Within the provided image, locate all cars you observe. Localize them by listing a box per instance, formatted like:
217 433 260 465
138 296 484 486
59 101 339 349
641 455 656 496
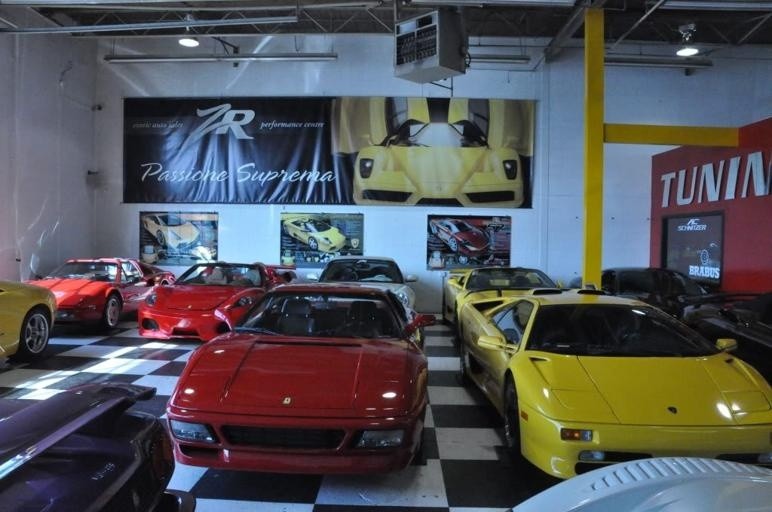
165 277 440 479
428 216 509 268
0 279 57 359
282 216 361 266
0 373 196 512
140 212 216 264
138 262 286 343
308 255 419 310
350 116 526 209
25 258 176 333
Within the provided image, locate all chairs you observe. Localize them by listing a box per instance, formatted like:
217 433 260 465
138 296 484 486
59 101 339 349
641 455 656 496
204 269 227 284
369 266 387 276
511 276 531 286
245 262 268 285
271 298 317 334
472 274 490 288
334 301 385 338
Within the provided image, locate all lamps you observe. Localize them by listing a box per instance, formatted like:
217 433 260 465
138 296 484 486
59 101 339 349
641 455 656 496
471 55 530 63
675 24 699 57
178 14 200 47
604 59 711 69
100 55 339 62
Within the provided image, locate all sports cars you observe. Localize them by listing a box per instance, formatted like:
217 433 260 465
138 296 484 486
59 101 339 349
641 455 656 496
461 285 772 483
672 291 772 387
567 267 708 319
442 265 563 331
